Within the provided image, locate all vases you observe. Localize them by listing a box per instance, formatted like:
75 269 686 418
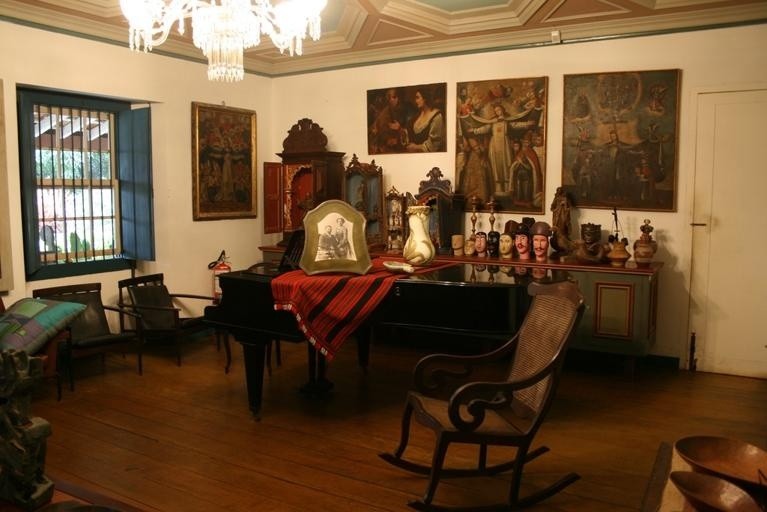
402 206 435 266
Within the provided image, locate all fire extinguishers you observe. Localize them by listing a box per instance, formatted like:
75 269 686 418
208 249 231 305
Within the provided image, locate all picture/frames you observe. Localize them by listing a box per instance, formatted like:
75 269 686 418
562 69 682 212
454 76 549 215
192 100 257 221
297 198 372 276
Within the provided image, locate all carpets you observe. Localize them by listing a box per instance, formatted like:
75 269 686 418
639 441 696 511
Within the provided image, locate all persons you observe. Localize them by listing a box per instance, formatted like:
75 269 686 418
197 107 253 212
388 88 445 152
455 80 543 211
499 234 515 258
334 217 354 260
565 73 674 210
475 232 487 256
579 223 601 253
550 187 572 237
318 225 339 261
475 263 548 283
513 223 533 259
369 88 417 154
529 222 551 262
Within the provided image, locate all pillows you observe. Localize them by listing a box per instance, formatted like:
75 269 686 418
0 298 88 356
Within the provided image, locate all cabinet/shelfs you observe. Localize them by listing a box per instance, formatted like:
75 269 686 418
264 118 347 246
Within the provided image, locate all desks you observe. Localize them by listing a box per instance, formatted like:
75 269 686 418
258 245 664 357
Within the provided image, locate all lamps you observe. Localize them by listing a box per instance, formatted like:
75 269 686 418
123 0 327 84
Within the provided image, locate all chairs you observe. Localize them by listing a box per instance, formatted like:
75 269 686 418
377 282 587 510
32 282 148 390
0 291 67 400
118 273 223 365
